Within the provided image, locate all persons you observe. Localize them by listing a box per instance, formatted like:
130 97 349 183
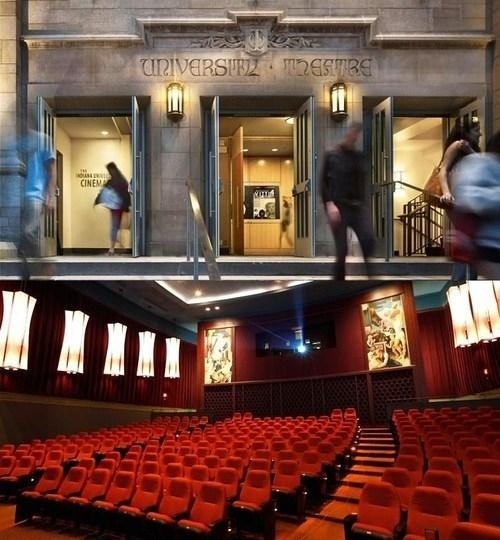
280 195 293 248
0 102 56 258
104 162 130 255
255 202 275 219
321 120 378 282
440 117 500 280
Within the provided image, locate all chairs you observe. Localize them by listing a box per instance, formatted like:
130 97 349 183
1 407 500 540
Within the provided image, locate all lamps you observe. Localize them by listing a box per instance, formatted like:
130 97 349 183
164 337 182 379
0 290 38 373
446 278 500 350
102 323 128 376
136 330 157 379
56 310 90 375
166 83 185 121
330 83 347 122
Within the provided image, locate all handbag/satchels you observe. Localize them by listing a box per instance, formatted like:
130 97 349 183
423 167 454 210
120 211 131 230
